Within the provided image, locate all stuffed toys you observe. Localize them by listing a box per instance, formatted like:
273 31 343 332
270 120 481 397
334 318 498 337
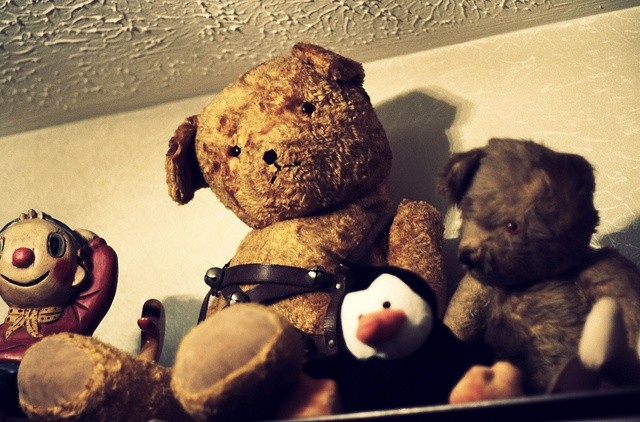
1 209 119 420
292 244 520 421
18 43 444 420
446 136 640 394
